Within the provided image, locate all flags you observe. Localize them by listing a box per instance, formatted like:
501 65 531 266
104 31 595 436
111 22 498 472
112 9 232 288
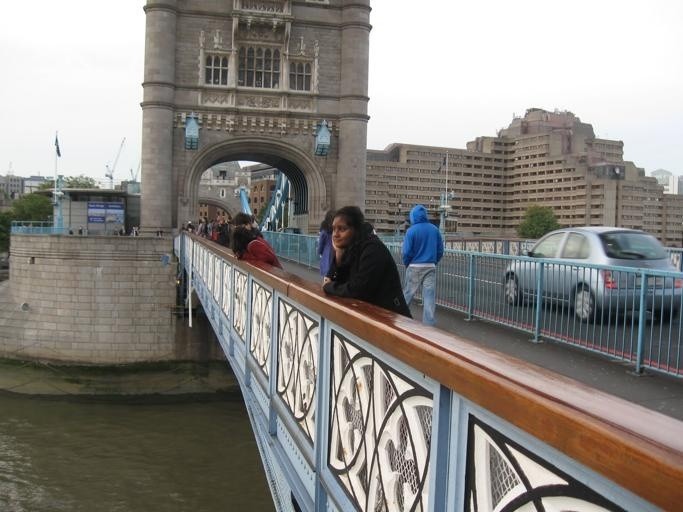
436 157 446 173
55 137 62 158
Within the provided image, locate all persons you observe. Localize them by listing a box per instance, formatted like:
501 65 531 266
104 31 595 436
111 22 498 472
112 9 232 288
402 205 444 326
322 205 412 319
318 211 336 281
181 213 282 269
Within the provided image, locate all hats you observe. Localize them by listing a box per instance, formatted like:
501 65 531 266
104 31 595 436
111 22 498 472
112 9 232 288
227 212 255 226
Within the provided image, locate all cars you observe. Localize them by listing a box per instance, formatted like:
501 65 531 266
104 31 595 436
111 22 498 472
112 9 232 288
274 227 307 253
501 226 683 322
